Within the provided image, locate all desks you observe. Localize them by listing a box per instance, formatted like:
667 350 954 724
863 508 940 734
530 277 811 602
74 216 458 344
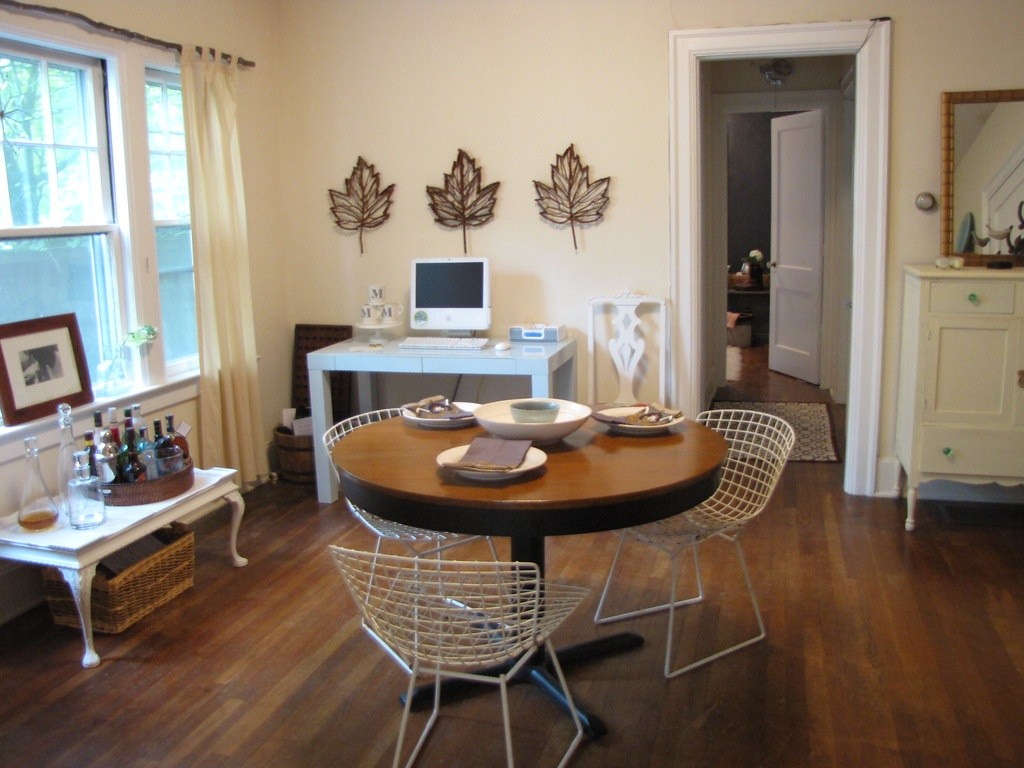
306 336 578 504
331 403 729 741
328 545 584 767
0 467 248 668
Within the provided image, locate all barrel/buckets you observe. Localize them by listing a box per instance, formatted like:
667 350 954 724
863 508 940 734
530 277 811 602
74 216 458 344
273 426 316 483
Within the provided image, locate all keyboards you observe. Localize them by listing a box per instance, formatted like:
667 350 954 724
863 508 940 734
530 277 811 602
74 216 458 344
399 337 489 350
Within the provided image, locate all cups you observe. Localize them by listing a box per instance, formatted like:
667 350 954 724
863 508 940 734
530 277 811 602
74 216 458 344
144 447 184 479
360 285 403 325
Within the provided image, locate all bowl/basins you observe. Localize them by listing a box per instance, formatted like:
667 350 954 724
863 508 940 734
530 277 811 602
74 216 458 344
473 397 593 445
510 400 560 423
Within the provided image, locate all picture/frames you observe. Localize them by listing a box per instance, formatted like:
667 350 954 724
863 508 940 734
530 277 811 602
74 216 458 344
0 313 94 426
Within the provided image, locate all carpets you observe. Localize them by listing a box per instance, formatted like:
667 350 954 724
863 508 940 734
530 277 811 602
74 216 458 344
705 399 842 463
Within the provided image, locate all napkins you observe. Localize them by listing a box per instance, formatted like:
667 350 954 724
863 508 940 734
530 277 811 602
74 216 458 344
590 404 682 425
401 395 473 420
442 436 532 471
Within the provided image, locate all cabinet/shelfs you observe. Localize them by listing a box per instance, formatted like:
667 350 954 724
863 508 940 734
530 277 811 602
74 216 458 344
893 264 1024 531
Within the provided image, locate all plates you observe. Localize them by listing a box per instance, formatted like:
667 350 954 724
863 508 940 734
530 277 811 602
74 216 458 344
607 407 685 433
436 444 547 480
402 401 482 428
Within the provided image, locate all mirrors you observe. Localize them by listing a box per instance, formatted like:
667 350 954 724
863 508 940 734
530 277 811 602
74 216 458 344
940 89 1024 267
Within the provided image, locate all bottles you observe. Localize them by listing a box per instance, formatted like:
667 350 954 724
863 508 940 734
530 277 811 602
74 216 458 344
57 405 80 515
65 453 106 529
17 437 59 530
84 403 190 484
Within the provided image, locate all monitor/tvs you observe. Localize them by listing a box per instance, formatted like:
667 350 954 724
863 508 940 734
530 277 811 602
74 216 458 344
410 257 492 338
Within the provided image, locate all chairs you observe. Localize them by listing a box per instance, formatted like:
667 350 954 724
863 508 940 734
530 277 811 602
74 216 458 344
323 408 514 675
586 295 671 409
594 408 797 679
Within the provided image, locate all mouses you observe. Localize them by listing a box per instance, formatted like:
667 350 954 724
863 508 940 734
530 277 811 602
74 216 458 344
495 343 510 351
